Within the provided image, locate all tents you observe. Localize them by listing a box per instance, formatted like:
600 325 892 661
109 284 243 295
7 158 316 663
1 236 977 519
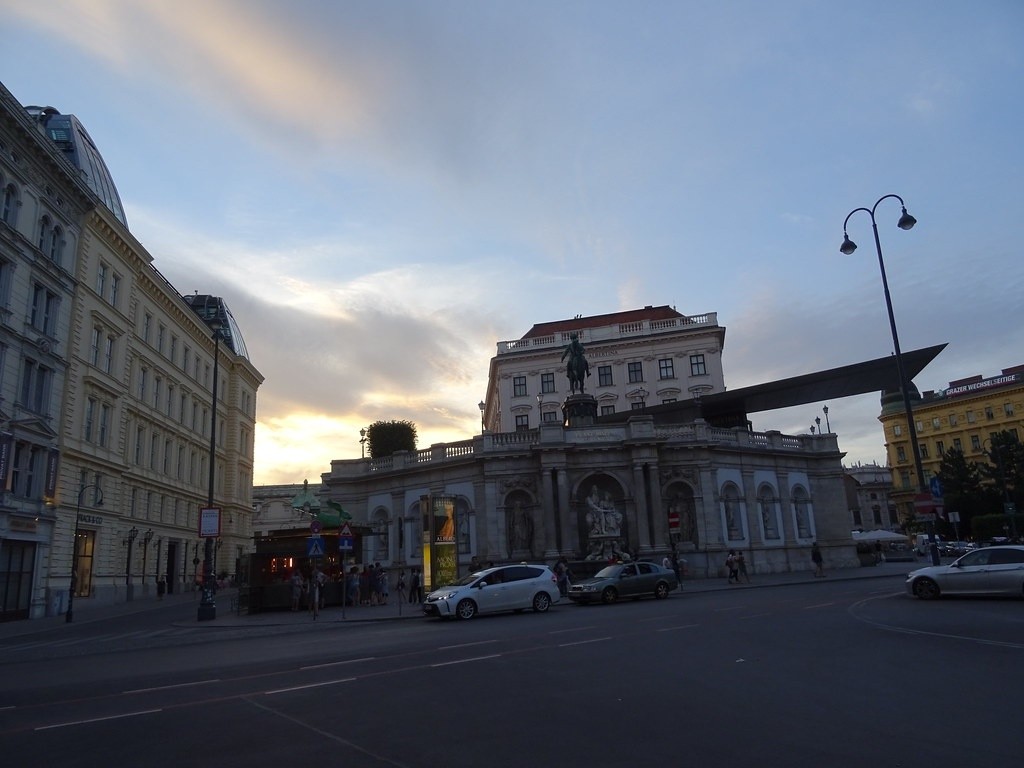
853 528 909 542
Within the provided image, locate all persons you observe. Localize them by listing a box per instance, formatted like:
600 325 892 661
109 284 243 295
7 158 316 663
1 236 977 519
290 561 423 611
609 555 618 566
554 557 575 597
811 541 826 578
919 541 933 562
873 540 884 568
726 550 753 585
631 548 641 564
585 485 623 535
561 334 591 380
671 554 687 591
468 556 482 572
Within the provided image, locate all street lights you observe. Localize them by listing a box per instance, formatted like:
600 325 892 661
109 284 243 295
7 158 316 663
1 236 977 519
66 486 105 623
360 428 366 458
197 312 226 622
536 392 544 422
478 401 486 434
837 192 943 565
810 405 830 435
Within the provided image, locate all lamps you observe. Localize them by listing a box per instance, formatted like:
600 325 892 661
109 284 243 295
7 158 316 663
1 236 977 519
129 526 138 540
145 529 154 543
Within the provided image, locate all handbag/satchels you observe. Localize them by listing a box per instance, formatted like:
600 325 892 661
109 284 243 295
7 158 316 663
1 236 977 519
554 565 561 576
726 560 729 566
401 582 404 589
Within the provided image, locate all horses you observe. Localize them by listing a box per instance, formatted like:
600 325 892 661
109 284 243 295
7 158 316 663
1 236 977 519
568 348 586 395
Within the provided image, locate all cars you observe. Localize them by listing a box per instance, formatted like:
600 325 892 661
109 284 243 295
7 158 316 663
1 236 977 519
566 560 678 604
423 564 562 620
912 534 1010 557
904 545 1024 601
